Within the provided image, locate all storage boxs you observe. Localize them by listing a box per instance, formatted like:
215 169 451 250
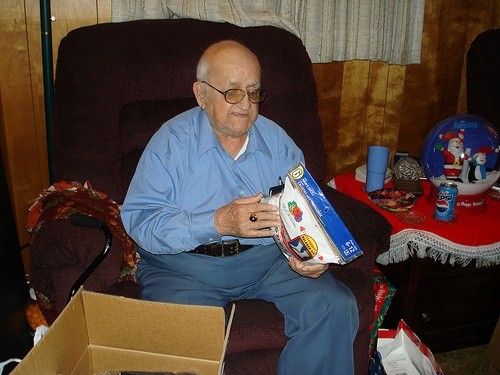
11 284 234 375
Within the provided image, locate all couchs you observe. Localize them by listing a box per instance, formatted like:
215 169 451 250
30 20 391 373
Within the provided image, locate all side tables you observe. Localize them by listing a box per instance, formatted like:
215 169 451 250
327 172 500 352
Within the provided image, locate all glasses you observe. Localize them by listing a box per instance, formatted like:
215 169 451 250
202 81 268 104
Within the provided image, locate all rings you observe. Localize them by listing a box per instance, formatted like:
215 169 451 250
249 212 257 223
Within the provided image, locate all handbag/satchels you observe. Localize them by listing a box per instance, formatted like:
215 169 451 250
376 319 443 375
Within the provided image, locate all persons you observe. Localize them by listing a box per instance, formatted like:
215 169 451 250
436 131 476 183
120 40 360 375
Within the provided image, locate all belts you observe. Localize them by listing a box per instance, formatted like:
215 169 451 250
185 240 254 258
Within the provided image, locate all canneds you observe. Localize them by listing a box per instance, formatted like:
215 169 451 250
435 182 458 221
